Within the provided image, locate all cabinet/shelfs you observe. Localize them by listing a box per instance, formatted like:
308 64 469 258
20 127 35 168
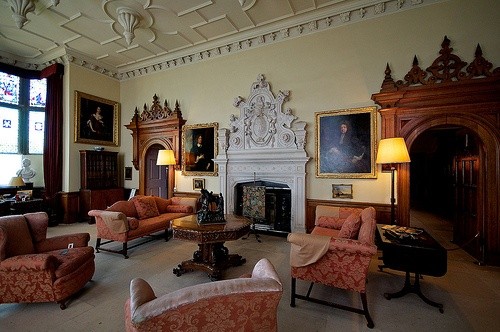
80 150 126 225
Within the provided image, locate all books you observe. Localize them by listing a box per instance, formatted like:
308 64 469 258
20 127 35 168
87 153 118 189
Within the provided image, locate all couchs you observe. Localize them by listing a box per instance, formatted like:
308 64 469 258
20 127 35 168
124 257 284 332
0 211 95 310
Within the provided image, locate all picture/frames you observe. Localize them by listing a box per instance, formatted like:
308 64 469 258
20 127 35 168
73 90 119 148
332 184 353 199
314 105 378 179
192 178 205 191
182 122 219 177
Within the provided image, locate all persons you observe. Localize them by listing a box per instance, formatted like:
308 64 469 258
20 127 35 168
86 105 106 137
16 158 38 183
189 134 211 170
326 120 369 174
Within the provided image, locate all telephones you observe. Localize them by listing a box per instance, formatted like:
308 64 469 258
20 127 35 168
384 229 411 244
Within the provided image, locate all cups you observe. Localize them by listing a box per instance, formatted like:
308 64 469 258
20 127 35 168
16 194 19 201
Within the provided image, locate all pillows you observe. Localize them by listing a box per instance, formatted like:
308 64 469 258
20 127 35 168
127 216 139 228
166 204 193 213
132 196 160 219
339 211 361 238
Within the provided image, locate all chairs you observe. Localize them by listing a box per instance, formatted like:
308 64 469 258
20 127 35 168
0 201 10 217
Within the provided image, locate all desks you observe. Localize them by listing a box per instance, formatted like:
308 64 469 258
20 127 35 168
170 213 253 282
0 198 44 216
376 222 448 314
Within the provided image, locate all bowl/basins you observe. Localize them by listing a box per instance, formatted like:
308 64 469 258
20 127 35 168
95 147 104 151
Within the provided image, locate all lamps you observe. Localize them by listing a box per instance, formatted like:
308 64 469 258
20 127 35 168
156 149 176 200
8 177 26 194
375 137 411 225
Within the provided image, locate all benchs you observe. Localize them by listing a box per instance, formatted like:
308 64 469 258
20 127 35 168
88 193 197 261
286 204 377 329
